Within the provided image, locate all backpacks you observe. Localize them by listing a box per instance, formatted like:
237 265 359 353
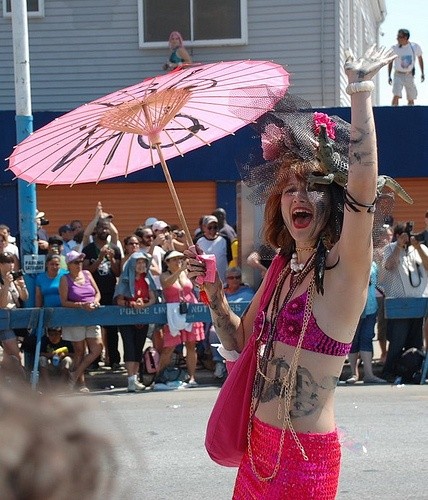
400 347 426 385
140 346 170 386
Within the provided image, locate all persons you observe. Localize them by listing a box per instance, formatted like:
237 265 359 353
163 32 192 72
0 201 427 392
0 373 119 499
387 29 425 106
183 41 398 500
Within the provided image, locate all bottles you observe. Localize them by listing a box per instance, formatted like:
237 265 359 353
52 353 60 367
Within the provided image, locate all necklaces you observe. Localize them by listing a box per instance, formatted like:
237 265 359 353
247 246 328 483
289 253 304 272
295 247 314 252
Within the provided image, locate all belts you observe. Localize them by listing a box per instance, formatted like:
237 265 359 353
395 70 412 74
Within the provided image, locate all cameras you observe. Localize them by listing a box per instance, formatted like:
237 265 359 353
405 221 426 245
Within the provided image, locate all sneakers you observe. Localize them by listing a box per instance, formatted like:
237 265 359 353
214 363 225 378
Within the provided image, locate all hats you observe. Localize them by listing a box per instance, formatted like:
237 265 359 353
152 221 169 232
36 209 45 218
228 83 413 258
202 215 218 225
101 212 112 219
58 225 76 234
66 251 85 264
164 251 185 262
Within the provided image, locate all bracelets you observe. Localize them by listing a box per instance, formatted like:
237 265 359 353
346 81 375 96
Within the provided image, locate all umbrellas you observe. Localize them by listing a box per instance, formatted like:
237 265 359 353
4 59 294 280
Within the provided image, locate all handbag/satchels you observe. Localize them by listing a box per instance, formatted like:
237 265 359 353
205 309 267 467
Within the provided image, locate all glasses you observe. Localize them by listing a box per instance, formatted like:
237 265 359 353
206 225 218 230
2 252 11 257
71 261 84 264
142 235 154 239
128 242 139 245
226 276 241 279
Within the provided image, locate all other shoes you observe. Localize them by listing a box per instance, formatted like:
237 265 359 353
66 351 206 393
346 376 358 384
364 376 387 383
380 375 396 383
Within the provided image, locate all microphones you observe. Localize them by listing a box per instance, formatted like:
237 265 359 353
10 268 25 281
